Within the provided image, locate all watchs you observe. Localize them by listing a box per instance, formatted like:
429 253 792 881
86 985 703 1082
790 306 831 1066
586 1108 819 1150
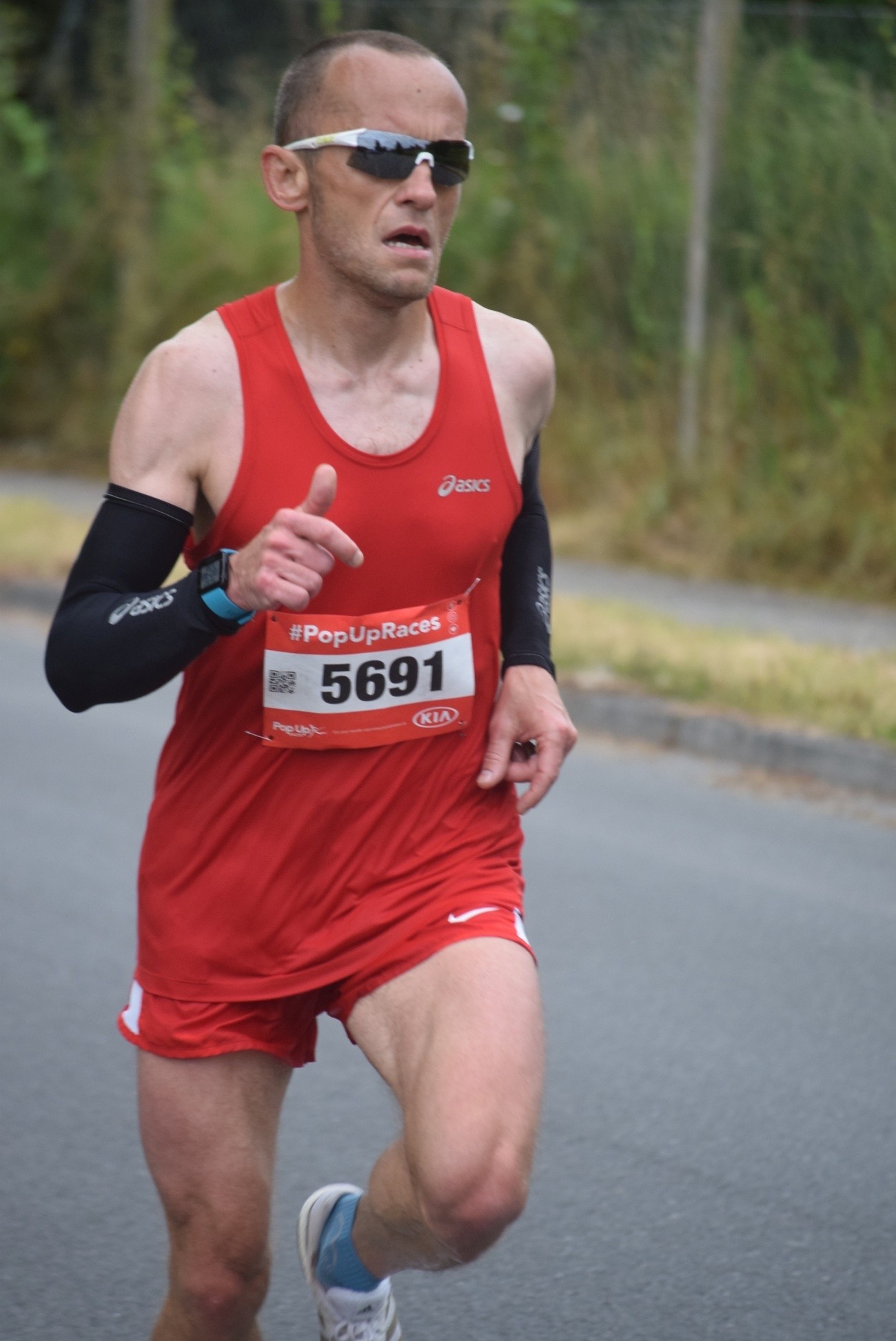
198 547 256 626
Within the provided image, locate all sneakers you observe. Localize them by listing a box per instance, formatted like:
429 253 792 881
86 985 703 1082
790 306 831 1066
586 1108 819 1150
297 1182 403 1341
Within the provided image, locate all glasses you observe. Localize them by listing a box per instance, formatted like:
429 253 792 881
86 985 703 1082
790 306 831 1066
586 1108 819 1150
284 128 474 186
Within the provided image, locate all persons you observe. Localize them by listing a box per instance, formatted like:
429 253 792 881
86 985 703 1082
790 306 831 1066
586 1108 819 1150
42 30 577 1341
